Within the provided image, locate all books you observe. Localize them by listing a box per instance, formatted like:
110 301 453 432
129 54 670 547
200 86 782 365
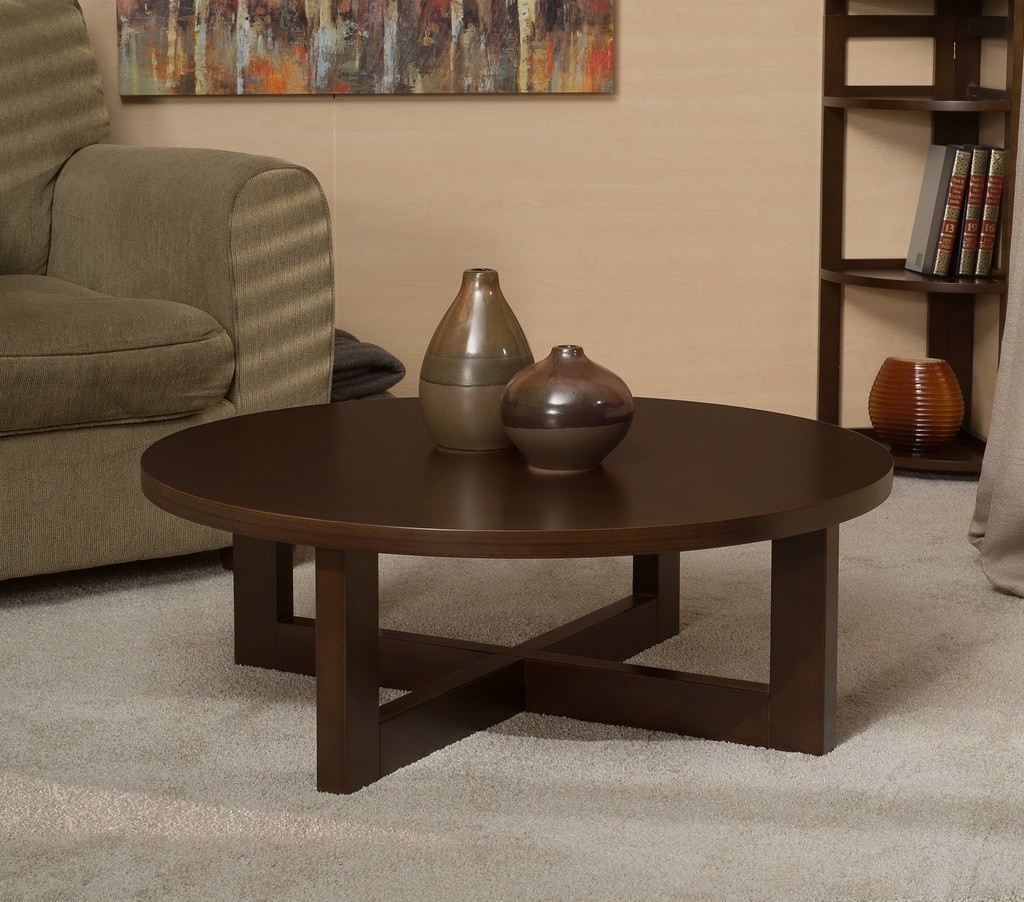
905 144 1006 279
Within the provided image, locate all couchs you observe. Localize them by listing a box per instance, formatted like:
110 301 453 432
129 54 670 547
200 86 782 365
0 0 336 584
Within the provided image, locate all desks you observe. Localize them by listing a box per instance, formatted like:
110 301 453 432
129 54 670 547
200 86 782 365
142 398 894 798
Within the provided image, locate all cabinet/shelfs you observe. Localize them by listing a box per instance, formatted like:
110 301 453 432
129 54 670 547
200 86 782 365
820 1 1023 473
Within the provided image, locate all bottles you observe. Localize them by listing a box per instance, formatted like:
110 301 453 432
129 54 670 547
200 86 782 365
420 268 535 451
501 345 635 471
